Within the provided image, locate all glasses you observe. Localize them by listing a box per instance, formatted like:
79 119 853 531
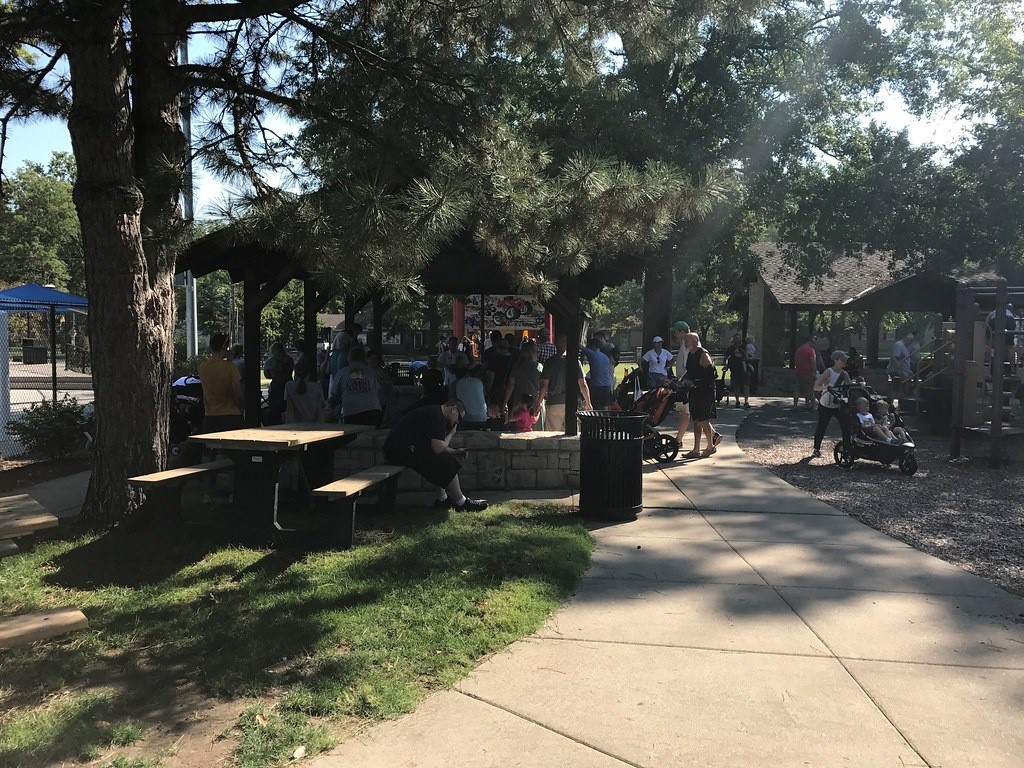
657 355 660 364
450 404 463 424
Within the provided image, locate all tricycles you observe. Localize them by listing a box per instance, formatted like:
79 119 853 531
715 369 730 407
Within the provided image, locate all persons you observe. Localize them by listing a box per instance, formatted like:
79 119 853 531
196 322 758 510
885 333 915 400
382 395 488 511
791 332 922 457
813 350 855 457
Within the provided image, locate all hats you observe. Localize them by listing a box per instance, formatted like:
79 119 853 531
653 336 664 342
831 350 850 360
669 321 690 331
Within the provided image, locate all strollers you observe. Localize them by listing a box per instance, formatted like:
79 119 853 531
615 367 644 411
625 378 693 463
828 383 919 476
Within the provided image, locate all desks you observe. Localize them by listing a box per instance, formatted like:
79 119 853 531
0 494 59 540
187 422 375 535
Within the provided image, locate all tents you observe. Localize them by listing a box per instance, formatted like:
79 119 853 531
0 284 88 312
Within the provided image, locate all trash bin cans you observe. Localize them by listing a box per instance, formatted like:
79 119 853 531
20 339 48 364
576 410 649 519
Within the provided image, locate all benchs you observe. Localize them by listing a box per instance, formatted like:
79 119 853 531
311 465 407 546
0 605 88 649
0 539 20 559
127 458 238 515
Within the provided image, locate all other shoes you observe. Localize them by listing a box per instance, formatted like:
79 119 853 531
891 436 903 444
682 451 700 458
712 433 723 445
813 449 822 457
446 495 487 512
668 439 682 448
701 446 716 457
